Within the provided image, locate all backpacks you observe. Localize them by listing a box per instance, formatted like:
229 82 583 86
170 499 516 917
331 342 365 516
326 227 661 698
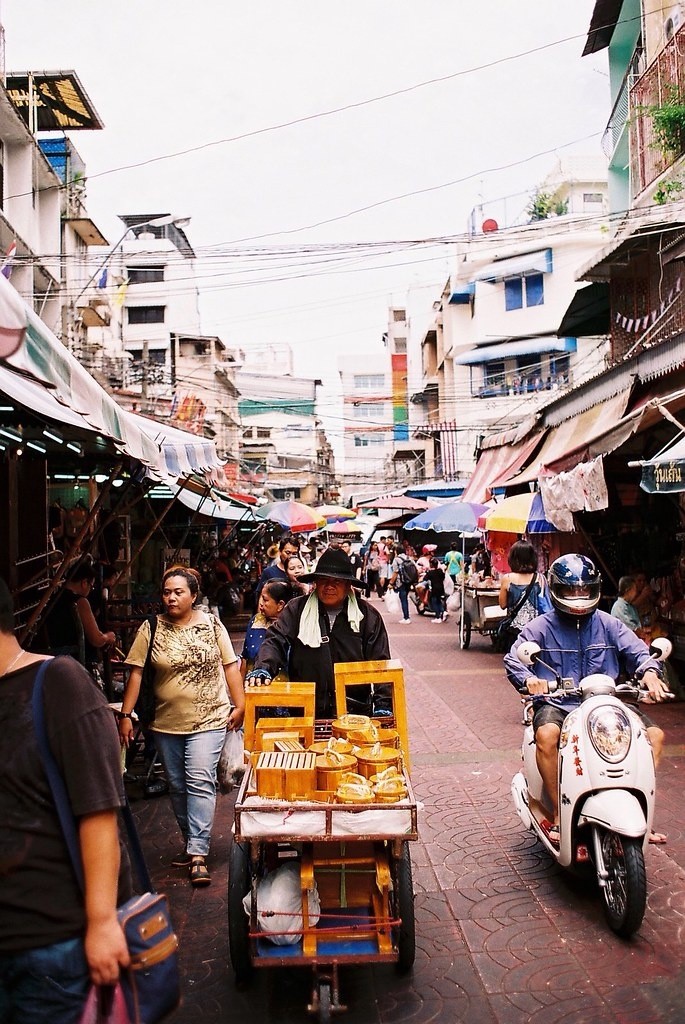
397 556 418 584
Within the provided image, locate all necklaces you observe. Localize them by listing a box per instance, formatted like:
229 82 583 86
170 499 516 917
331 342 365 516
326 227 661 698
3 650 25 675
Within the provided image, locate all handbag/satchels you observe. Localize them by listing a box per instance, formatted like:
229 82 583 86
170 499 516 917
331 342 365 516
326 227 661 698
443 571 454 595
217 728 246 795
133 615 157 724
495 617 515 652
117 892 182 1024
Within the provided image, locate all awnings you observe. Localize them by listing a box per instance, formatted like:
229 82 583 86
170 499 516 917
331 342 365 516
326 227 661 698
460 379 685 506
0 269 265 636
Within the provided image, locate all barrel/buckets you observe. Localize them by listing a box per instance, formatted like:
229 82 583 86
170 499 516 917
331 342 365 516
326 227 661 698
309 737 358 791
332 715 401 787
336 785 408 804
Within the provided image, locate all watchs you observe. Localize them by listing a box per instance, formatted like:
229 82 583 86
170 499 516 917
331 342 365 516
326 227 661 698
118 712 131 720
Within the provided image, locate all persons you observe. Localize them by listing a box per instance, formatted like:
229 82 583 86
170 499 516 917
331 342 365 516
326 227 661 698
117 563 245 884
502 553 669 844
203 536 491 625
42 561 116 671
0 583 131 1024
611 572 656 632
243 546 393 727
499 541 552 726
238 578 304 683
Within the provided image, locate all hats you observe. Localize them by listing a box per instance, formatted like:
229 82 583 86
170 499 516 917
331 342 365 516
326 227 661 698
296 548 369 589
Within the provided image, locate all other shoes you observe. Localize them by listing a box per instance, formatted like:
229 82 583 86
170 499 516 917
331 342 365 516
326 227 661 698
638 693 656 704
191 860 211 882
431 617 442 623
399 619 410 624
172 848 193 865
443 611 448 621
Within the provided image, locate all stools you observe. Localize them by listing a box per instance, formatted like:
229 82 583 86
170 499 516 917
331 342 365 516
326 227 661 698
255 716 317 757
333 659 410 775
241 680 315 768
294 840 393 957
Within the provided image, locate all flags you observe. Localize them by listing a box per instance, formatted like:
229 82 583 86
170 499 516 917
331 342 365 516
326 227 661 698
616 278 681 333
99 268 107 289
114 281 126 304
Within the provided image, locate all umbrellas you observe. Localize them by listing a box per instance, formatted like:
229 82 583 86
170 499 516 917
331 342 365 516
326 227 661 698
477 489 565 572
257 494 434 543
462 519 493 650
403 501 492 650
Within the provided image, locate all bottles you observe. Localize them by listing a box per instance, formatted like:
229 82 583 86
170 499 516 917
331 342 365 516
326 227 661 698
472 572 479 588
485 576 491 588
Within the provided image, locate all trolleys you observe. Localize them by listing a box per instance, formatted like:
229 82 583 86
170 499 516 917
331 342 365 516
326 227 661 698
454 582 508 650
227 692 418 1024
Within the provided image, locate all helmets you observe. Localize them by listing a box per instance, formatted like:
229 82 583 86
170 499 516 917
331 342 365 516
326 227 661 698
547 554 601 615
422 544 437 553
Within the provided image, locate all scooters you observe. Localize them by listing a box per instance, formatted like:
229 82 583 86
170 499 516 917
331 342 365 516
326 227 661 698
415 580 450 618
518 637 676 936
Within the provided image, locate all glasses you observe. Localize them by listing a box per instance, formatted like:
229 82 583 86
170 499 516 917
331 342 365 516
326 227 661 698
87 580 95 592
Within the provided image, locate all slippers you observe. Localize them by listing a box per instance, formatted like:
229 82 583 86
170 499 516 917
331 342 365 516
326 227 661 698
648 829 666 843
549 827 561 845
